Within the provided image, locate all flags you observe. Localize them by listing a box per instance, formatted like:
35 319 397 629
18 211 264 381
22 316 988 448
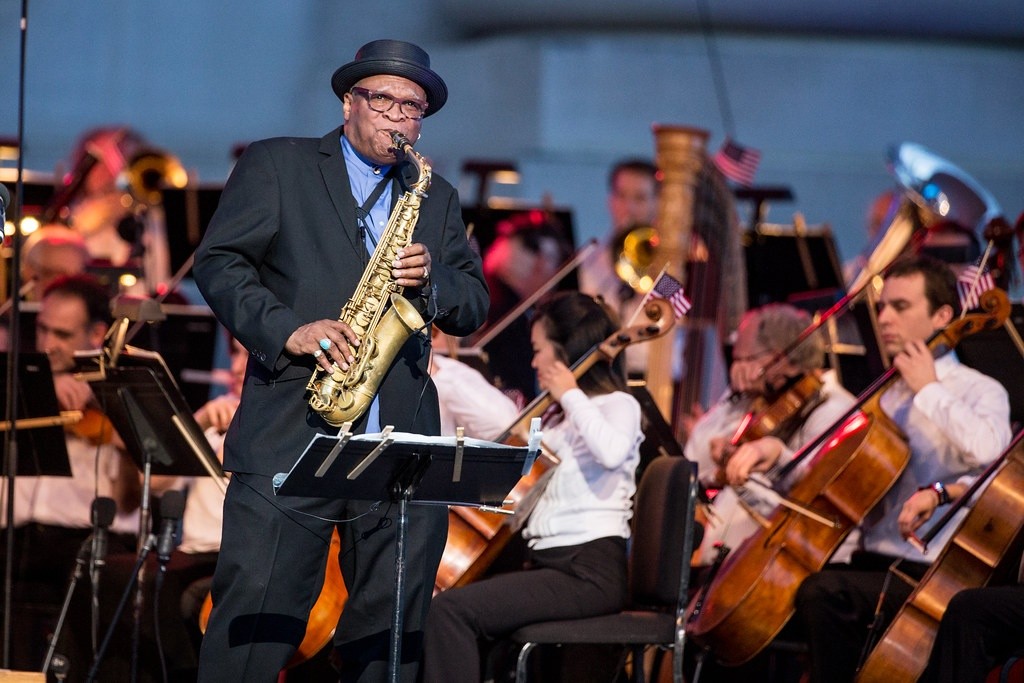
645 272 694 319
956 256 996 311
713 137 760 186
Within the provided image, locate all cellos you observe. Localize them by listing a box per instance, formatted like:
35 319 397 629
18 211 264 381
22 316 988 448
686 285 1012 664
847 423 1024 683
200 526 349 665
430 292 680 604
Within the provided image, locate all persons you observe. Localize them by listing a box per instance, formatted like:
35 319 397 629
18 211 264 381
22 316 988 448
199 39 490 683
0 129 254 675
682 303 859 592
575 160 706 384
423 291 646 683
428 353 520 442
726 257 1012 683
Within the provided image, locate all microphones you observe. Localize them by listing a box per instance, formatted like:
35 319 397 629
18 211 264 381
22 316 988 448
89 497 117 593
156 490 186 565
0 182 11 245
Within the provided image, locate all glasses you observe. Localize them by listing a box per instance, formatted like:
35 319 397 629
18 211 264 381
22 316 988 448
349 86 429 119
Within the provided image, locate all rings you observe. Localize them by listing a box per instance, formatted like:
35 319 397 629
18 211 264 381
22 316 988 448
314 349 322 358
422 266 429 279
320 338 331 349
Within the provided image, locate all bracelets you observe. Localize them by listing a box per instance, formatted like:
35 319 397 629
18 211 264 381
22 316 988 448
930 481 951 506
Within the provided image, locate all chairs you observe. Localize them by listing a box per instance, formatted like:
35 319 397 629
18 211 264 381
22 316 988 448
486 451 699 683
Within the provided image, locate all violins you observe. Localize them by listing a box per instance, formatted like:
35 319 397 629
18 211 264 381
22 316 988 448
66 393 120 448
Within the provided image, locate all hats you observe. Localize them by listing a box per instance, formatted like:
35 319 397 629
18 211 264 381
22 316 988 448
332 39 447 119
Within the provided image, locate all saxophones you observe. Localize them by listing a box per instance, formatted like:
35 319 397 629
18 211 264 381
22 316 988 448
306 125 436 428
